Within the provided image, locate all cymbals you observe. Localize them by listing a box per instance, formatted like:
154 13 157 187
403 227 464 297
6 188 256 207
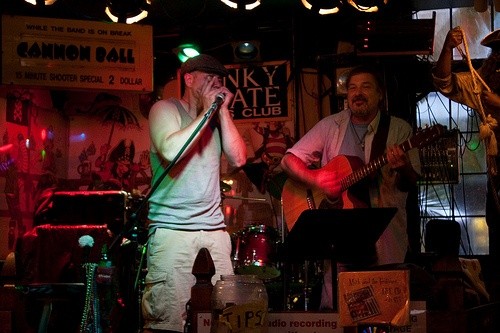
267 167 317 201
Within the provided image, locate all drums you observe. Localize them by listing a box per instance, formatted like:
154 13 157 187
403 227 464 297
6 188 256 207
234 223 283 280
229 228 248 261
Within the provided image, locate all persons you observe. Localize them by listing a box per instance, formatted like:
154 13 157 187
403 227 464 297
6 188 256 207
281 66 422 310
432 26 500 254
142 54 248 333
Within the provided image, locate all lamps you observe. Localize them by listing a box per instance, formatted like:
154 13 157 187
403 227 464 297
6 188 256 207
98 0 396 64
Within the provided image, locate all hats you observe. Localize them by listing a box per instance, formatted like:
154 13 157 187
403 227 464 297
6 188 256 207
180 54 231 75
480 30 500 47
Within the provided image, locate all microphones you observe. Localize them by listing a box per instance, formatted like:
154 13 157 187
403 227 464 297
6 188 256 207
215 93 225 105
78 235 94 264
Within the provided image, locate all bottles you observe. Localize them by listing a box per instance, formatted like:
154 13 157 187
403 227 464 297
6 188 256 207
212 274 269 314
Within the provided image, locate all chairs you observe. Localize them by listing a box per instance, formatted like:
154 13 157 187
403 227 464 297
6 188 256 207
13 223 117 333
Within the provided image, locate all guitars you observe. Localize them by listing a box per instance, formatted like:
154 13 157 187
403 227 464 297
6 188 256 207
281 122 448 234
